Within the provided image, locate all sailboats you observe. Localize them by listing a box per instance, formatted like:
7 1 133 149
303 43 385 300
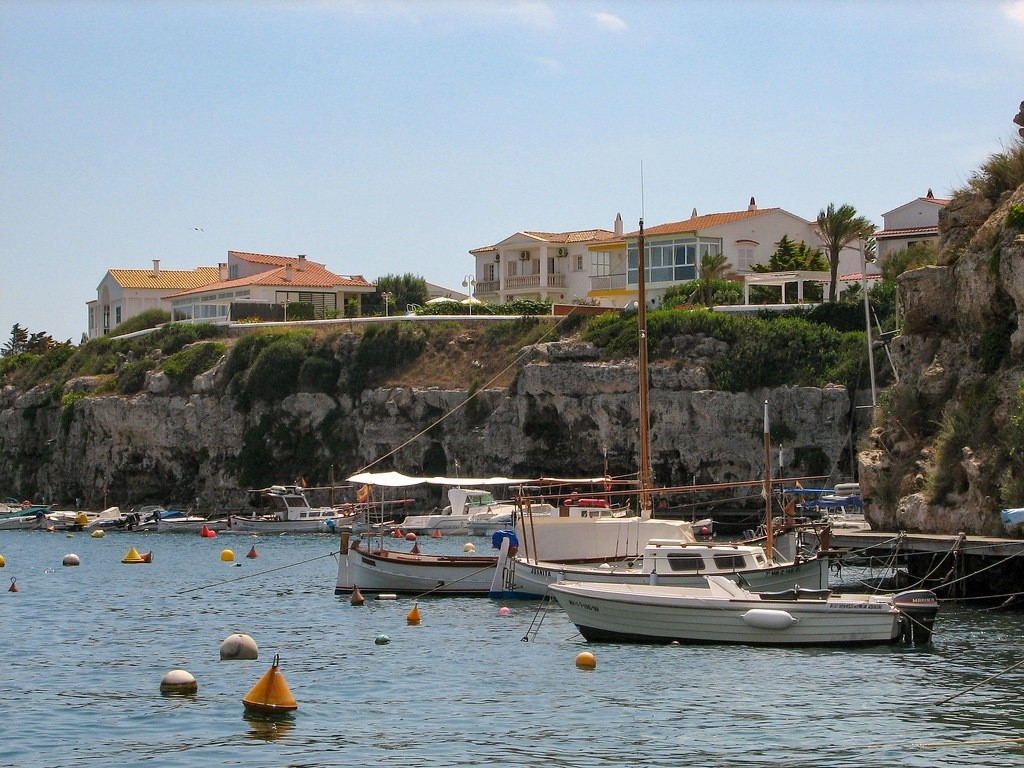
330 216 864 602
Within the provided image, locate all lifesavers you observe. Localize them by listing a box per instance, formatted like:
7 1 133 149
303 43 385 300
492 530 519 558
22 501 31 510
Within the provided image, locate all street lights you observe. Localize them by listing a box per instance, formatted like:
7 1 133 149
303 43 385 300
381 291 394 317
462 274 477 316
282 299 290 322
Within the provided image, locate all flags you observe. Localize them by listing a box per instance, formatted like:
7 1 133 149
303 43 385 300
356 484 369 503
302 479 306 488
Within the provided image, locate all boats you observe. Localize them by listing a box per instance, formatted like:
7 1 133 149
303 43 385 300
0 496 231 535
546 399 942 650
228 484 368 537
